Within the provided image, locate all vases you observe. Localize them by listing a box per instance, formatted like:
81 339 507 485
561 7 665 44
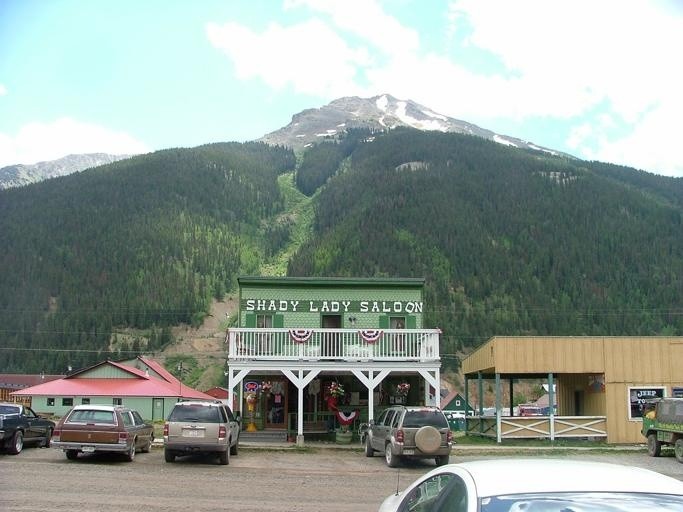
326 397 337 410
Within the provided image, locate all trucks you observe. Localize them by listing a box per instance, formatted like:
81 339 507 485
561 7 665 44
639 394 683 462
518 402 545 418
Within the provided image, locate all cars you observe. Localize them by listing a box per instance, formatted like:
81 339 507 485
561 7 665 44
374 456 683 512
53 402 156 462
0 402 56 455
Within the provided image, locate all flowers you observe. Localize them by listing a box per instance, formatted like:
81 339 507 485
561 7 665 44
329 382 345 399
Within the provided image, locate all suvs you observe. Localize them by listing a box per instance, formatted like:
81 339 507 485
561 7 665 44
362 402 456 468
161 396 243 468
